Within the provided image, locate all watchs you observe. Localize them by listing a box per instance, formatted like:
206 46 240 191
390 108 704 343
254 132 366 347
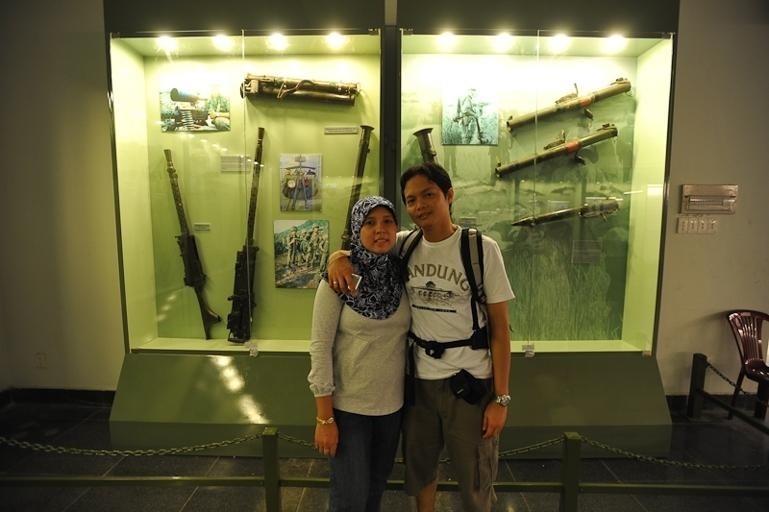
492 391 513 408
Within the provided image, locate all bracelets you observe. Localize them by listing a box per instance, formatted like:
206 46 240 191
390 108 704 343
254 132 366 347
315 415 335 424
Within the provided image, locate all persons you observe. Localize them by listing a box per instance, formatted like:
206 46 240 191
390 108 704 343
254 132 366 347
316 158 517 510
274 224 328 274
302 192 410 512
458 86 484 145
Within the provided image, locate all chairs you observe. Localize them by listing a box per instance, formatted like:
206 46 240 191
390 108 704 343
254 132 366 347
726 310 769 421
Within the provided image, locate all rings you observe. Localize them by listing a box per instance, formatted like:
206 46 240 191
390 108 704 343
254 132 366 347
333 281 338 284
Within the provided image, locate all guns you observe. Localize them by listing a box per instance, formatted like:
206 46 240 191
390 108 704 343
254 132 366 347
164 149 220 339
228 127 264 343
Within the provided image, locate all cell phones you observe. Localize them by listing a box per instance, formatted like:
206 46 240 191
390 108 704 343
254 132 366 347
348 274 362 290
